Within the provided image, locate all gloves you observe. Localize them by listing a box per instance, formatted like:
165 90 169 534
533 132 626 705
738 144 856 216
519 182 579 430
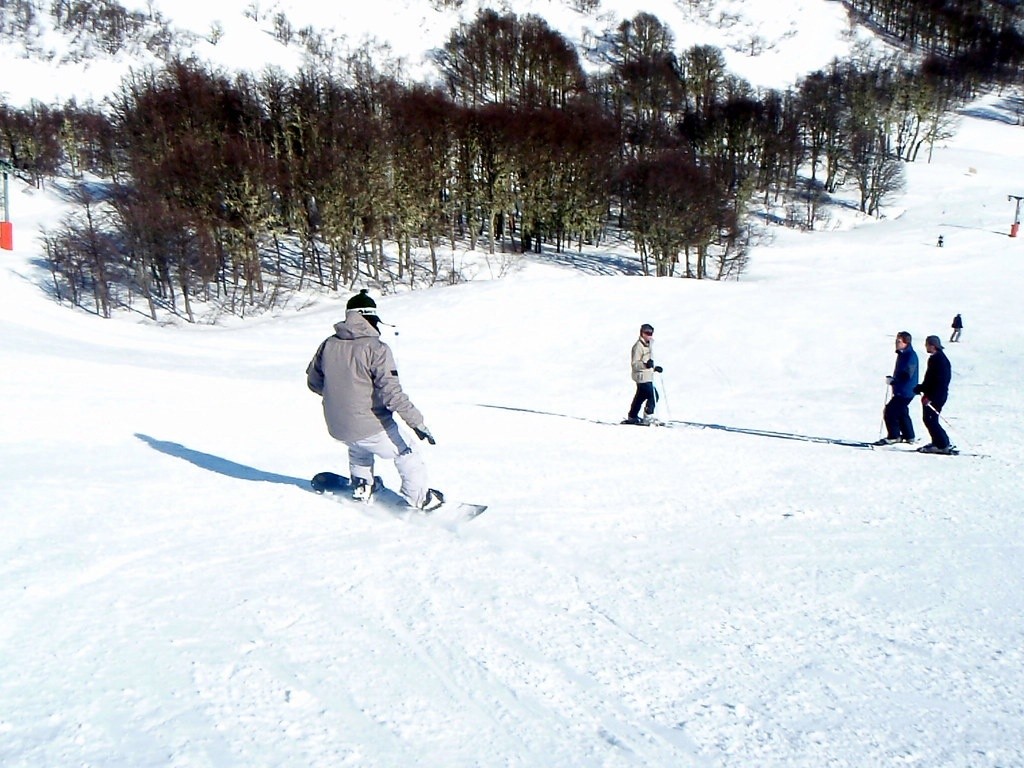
921 396 931 405
886 377 894 385
646 359 653 368
412 422 436 446
913 384 922 394
654 366 662 373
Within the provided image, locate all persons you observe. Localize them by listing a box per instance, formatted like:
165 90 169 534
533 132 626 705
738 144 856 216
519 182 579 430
628 324 664 426
913 335 951 453
949 314 963 342
305 289 444 513
879 331 919 445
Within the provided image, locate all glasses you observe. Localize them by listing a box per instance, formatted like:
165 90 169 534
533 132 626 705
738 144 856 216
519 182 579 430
643 330 653 336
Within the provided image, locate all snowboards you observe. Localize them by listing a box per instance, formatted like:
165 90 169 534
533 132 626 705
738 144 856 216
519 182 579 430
309 470 488 526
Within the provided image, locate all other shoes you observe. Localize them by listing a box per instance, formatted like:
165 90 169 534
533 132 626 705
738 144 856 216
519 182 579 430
955 340 959 342
949 340 954 342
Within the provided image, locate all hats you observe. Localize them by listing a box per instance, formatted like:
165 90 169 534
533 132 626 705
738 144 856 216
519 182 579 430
925 336 945 349
898 331 912 343
345 289 395 329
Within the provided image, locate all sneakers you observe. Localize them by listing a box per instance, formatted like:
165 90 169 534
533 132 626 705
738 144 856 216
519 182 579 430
903 437 915 445
352 475 385 502
918 444 949 454
628 414 643 425
875 436 902 445
398 490 444 510
643 409 664 426
